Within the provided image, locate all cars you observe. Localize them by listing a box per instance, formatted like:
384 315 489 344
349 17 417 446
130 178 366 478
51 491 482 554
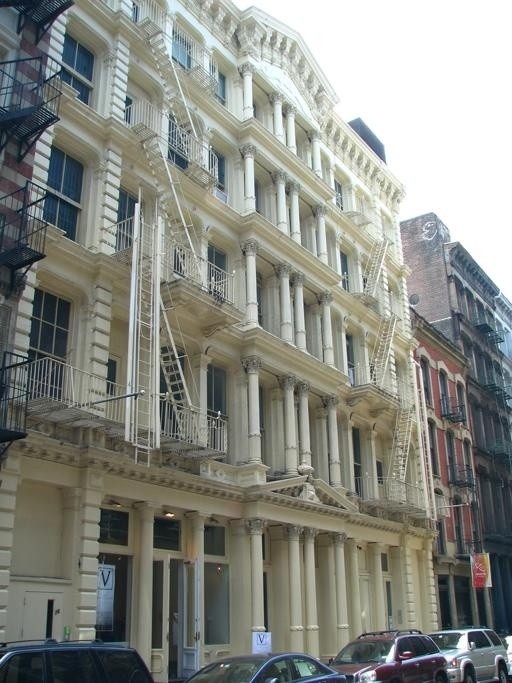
184 652 347 683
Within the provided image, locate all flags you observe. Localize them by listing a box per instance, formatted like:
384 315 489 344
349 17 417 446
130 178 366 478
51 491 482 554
469 552 495 589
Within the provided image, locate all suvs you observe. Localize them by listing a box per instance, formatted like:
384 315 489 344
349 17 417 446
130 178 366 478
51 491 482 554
322 625 512 682
0 638 156 683
